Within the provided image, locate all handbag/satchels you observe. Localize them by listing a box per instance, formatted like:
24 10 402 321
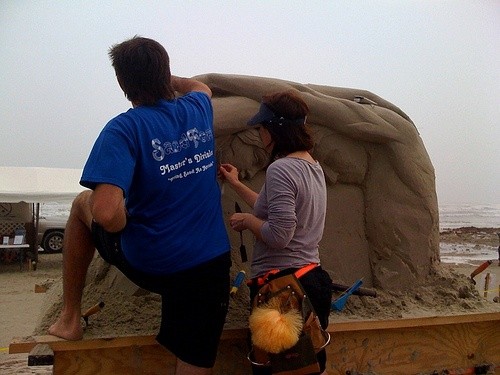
247 273 331 375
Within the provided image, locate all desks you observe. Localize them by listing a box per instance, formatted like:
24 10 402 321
0 244 29 271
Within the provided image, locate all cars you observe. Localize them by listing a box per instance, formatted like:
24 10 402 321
35 216 69 253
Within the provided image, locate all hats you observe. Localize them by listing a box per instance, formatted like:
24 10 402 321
247 92 309 127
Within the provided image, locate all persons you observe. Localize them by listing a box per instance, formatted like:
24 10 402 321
48 35 234 375
217 90 332 375
